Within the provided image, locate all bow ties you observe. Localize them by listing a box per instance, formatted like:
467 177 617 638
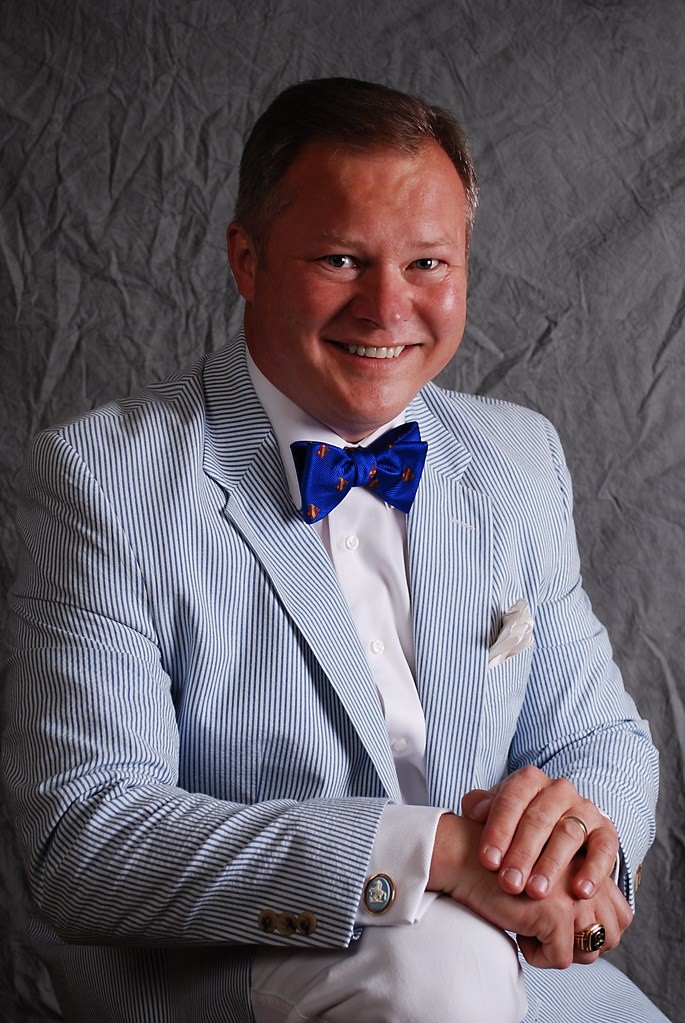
290 422 429 525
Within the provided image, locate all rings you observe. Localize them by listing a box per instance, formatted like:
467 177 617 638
561 816 588 841
574 923 605 953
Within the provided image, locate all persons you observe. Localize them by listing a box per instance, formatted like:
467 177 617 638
0 77 670 1023
